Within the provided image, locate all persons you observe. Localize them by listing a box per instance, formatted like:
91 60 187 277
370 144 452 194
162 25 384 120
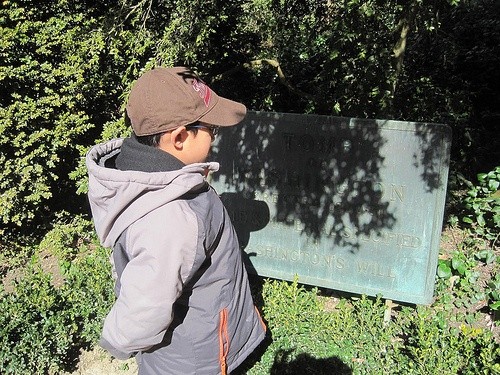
85 67 266 375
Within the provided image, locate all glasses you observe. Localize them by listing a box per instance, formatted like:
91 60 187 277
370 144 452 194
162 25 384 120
185 125 220 135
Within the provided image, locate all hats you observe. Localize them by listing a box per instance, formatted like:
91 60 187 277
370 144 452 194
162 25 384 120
127 67 246 136
